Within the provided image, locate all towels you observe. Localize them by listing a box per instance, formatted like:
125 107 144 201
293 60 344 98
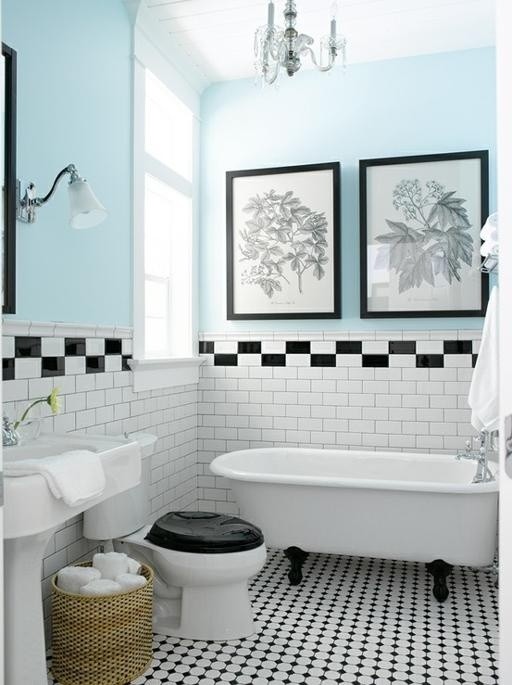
56 546 150 602
465 276 510 441
1 450 115 510
477 210 499 273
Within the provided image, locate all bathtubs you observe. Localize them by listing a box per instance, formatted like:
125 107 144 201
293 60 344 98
207 446 500 603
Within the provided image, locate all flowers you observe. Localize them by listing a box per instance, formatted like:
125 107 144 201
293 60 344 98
14 386 65 427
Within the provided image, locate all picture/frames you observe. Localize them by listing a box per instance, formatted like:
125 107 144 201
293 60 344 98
354 148 491 319
221 158 345 322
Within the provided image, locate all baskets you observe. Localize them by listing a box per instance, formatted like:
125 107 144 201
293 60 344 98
52 560 154 685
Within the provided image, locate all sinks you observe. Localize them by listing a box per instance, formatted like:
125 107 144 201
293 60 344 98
1 430 142 540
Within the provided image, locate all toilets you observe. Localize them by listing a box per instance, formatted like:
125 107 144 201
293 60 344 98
81 430 269 642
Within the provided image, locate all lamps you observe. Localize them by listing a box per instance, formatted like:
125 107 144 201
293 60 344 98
247 0 348 86
15 158 115 237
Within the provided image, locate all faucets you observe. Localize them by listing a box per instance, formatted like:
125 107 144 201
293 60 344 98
0 415 19 446
454 440 485 462
